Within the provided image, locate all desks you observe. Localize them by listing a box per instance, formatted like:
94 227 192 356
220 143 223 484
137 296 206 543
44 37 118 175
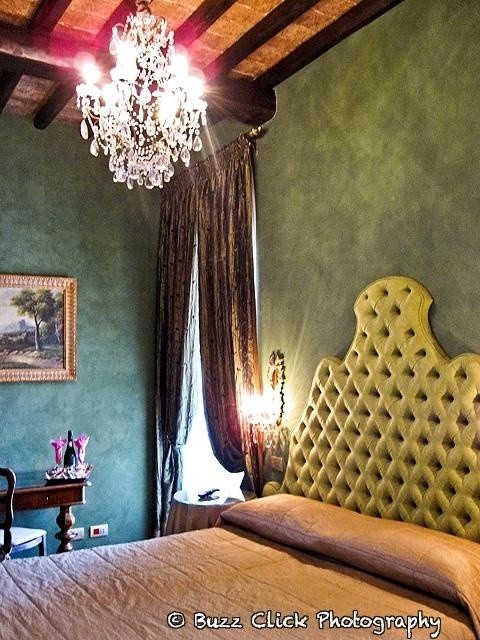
173 489 257 532
0 480 93 554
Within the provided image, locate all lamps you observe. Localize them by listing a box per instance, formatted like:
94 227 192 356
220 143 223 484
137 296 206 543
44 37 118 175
68 9 279 190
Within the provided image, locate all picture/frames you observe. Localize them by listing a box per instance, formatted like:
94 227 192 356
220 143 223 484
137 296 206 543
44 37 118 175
0 274 79 383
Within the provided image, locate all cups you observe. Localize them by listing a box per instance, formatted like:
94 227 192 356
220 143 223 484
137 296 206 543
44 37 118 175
54 447 63 465
77 446 86 465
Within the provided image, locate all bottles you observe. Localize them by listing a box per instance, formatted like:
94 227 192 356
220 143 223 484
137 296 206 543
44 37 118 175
63 430 76 468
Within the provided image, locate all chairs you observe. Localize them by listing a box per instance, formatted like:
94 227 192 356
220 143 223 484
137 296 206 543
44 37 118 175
0 466 48 561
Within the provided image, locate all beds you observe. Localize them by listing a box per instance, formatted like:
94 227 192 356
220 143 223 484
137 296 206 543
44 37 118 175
0 274 480 640
